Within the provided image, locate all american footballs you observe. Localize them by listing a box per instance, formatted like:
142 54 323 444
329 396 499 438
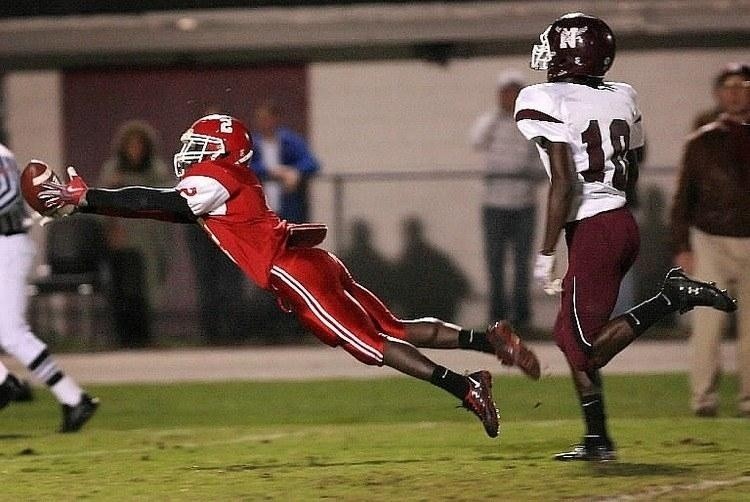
21 161 61 215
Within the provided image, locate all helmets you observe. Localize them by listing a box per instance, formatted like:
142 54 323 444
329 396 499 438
174 115 253 178
530 12 614 83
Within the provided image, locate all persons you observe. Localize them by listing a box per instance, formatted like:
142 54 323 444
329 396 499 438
669 65 750 416
241 107 321 347
513 8 740 462
0 140 100 433
38 113 539 438
179 102 246 346
94 119 175 349
470 69 552 344
686 59 738 130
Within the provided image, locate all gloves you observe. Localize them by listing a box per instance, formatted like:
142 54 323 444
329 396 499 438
34 213 65 226
37 166 89 209
535 250 562 296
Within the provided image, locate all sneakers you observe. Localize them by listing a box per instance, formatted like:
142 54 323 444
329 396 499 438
459 370 499 438
0 375 22 408
486 321 541 380
59 394 99 434
661 267 736 312
552 436 614 462
